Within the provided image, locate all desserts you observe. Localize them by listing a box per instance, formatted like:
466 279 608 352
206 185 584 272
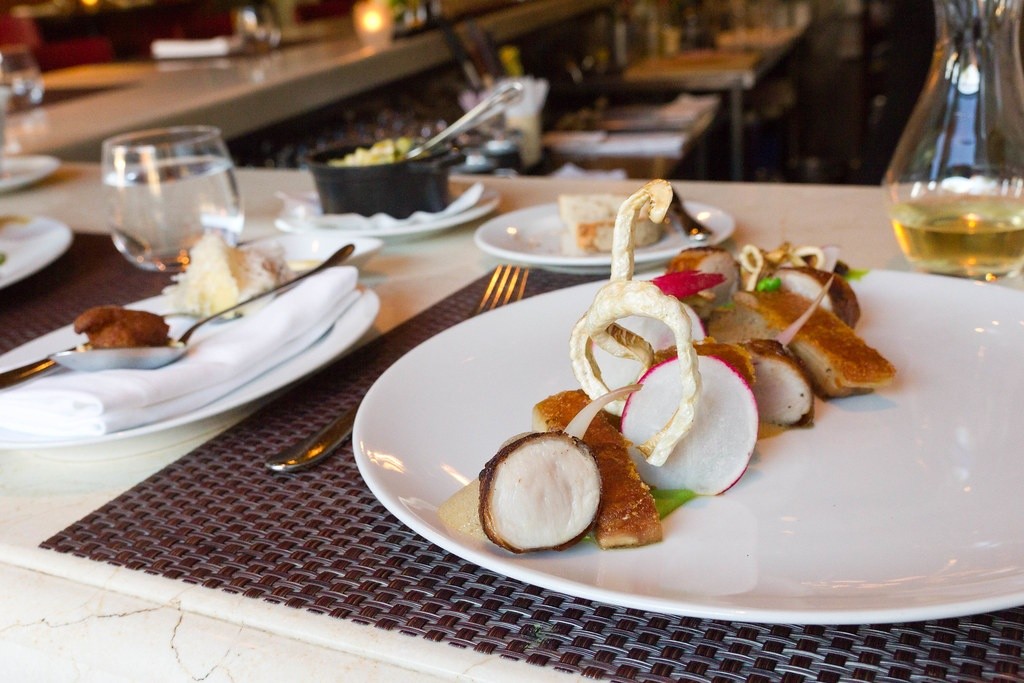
557 186 663 253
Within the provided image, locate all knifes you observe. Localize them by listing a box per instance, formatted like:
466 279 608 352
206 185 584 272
670 189 712 240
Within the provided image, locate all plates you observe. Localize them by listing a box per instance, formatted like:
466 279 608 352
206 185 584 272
240 235 384 271
1 155 60 194
0 285 382 451
274 181 500 235
0 214 74 290
353 270 1024 625
474 201 736 274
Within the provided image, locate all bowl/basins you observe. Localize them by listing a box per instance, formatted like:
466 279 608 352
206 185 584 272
304 141 465 218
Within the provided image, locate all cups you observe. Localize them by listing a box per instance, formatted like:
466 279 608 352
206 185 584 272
230 4 281 55
100 124 245 273
0 44 45 111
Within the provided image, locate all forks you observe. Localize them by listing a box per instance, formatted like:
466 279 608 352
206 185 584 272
266 265 529 470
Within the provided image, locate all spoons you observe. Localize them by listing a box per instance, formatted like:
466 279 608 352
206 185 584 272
0 312 243 389
48 244 357 371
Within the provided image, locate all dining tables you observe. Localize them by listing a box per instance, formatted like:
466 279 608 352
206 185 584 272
0 0 1024 683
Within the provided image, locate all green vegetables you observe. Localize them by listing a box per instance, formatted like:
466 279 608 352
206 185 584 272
754 267 868 292
649 488 693 519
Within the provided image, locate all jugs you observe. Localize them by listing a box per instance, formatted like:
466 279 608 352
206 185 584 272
882 0 1024 279
